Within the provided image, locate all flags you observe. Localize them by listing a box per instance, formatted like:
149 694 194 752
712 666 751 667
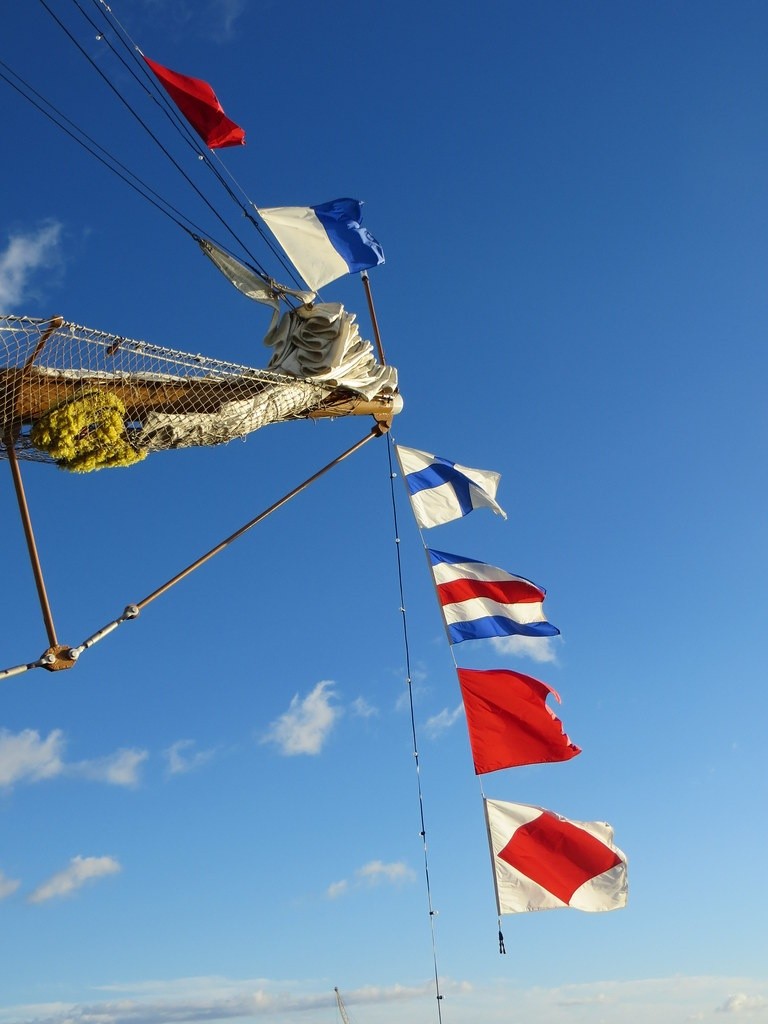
144 56 246 148
395 445 507 528
424 549 560 644
260 197 385 293
487 799 628 913
456 668 581 775
